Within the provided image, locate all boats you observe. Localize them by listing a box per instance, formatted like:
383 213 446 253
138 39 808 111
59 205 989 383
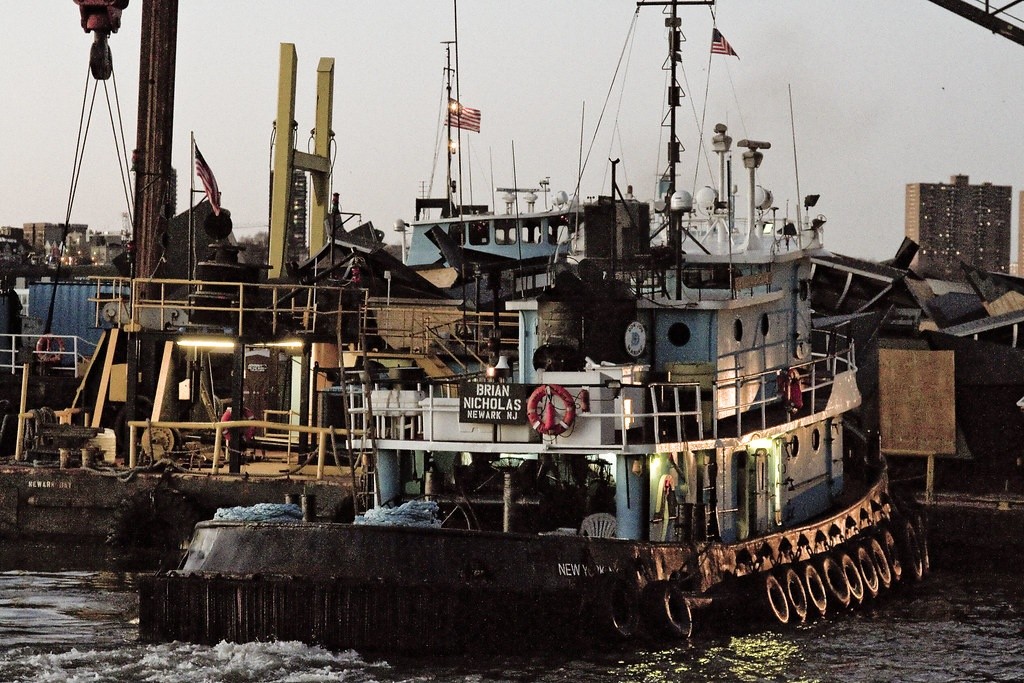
137 1 930 666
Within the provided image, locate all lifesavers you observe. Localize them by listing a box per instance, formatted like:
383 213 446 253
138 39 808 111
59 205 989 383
220 406 256 442
780 367 807 416
527 385 577 435
596 515 931 644
36 334 66 362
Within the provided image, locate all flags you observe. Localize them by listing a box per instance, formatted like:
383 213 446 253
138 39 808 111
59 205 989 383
194 143 222 217
712 28 740 59
445 99 481 133
